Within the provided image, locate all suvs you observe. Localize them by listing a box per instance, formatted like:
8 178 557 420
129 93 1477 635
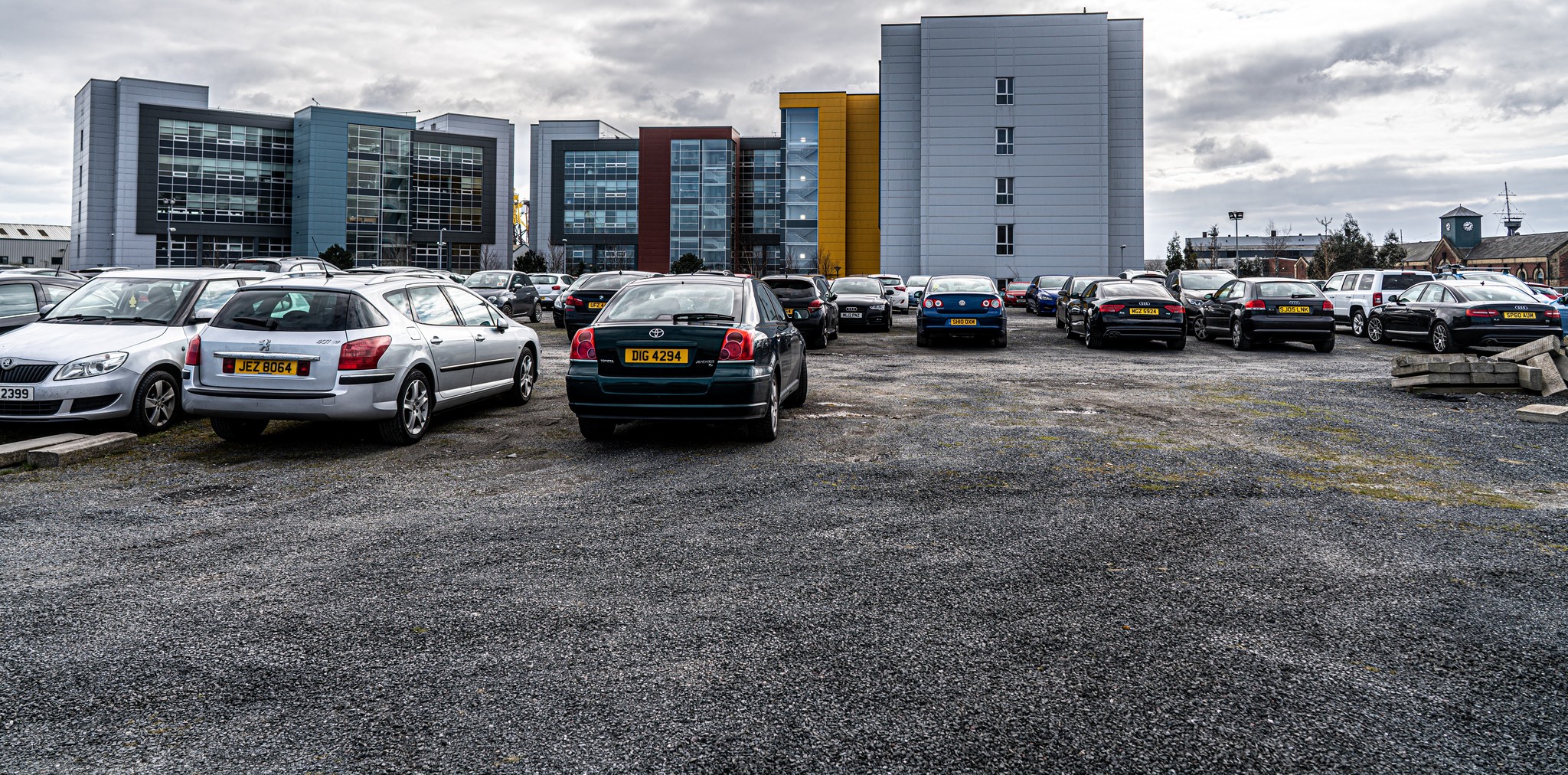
1118 268 1239 332
1321 268 1437 339
1001 281 1032 307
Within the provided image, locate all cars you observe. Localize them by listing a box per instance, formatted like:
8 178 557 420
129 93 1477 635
0 256 542 446
1192 277 1335 352
564 276 807 442
1367 267 1568 354
1055 275 1187 350
524 268 909 348
914 273 1008 348
905 275 933 307
1026 274 1074 316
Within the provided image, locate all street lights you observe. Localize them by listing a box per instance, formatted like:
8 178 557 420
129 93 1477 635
562 239 567 274
162 197 176 268
1228 210 1243 279
1120 245 1127 273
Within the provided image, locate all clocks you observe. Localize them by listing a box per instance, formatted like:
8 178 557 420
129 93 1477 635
1445 222 1451 232
1462 221 1474 232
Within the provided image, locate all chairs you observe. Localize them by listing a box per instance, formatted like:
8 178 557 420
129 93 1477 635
54 291 70 304
1277 283 1291 297
708 298 725 314
1465 289 1512 301
135 286 177 319
632 296 681 316
1076 281 1086 292
912 281 918 285
1386 279 1413 288
1434 292 1449 302
542 278 549 283
971 283 984 289
485 278 494 285
608 279 622 288
891 280 897 285
938 283 949 292
